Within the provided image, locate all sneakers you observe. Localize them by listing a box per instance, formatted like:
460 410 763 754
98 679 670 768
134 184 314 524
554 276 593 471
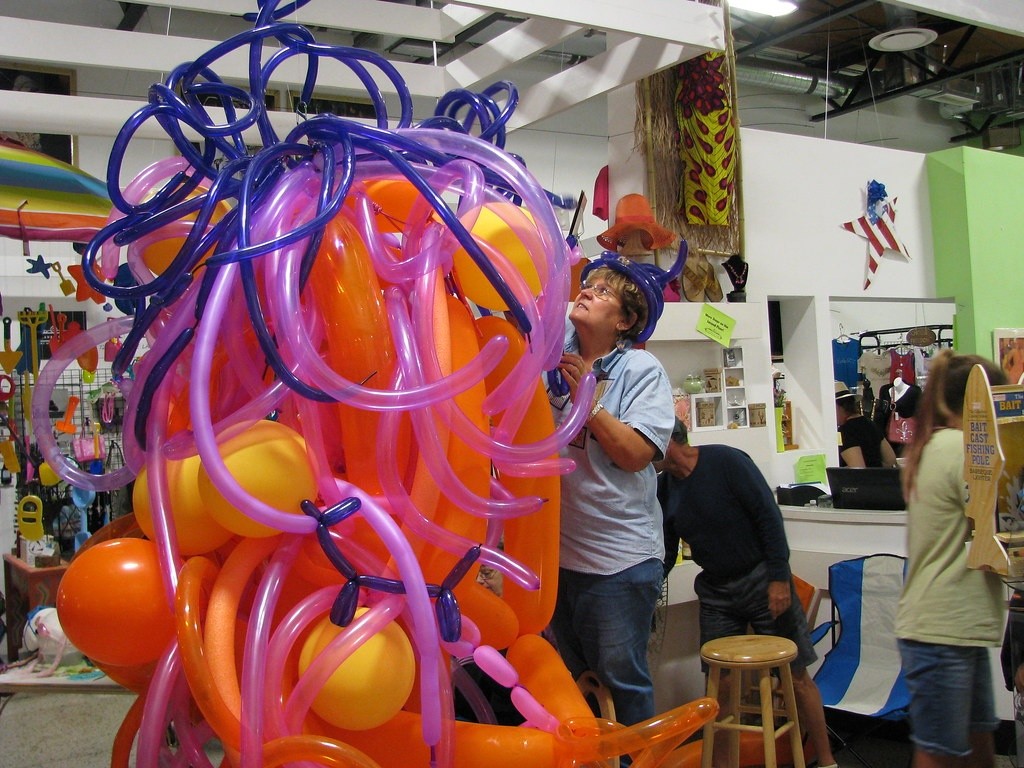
817 758 840 767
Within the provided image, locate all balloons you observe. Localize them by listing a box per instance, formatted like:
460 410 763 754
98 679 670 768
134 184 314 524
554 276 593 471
197 419 318 540
452 202 549 312
32 0 689 767
131 450 234 558
298 607 415 731
55 537 188 668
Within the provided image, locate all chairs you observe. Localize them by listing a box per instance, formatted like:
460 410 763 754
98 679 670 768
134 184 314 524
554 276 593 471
771 554 910 768
742 571 823 727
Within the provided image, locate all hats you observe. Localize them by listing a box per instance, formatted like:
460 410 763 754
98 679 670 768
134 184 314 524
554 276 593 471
596 193 677 253
835 381 863 403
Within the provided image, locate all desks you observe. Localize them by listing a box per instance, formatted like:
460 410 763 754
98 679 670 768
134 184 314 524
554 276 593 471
2 554 70 662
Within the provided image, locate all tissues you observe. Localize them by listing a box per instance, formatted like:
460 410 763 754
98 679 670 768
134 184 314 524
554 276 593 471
35 548 60 568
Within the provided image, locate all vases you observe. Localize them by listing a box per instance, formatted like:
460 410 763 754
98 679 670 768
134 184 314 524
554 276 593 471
776 408 785 452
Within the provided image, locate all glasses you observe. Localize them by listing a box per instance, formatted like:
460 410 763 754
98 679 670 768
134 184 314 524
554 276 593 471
581 282 624 306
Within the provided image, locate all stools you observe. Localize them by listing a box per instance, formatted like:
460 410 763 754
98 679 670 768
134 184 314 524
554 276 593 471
700 635 807 768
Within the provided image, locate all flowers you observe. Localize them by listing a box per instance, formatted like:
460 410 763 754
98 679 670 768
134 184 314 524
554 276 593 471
773 387 788 408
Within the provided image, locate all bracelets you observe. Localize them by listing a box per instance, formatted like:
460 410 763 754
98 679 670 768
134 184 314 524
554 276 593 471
587 401 603 421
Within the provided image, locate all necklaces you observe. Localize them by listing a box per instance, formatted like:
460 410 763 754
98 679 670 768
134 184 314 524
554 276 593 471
859 399 876 422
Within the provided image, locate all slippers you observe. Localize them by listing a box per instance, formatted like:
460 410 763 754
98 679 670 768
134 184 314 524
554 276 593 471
682 257 723 302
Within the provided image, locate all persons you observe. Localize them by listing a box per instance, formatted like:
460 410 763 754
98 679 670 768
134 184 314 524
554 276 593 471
834 381 896 468
652 414 838 768
896 350 1024 768
539 264 676 768
855 379 892 434
878 376 921 457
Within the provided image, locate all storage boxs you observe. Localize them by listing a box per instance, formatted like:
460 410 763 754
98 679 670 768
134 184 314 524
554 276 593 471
18 534 55 565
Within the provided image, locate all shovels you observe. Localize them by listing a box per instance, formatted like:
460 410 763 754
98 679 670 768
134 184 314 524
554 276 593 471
51 261 77 296
72 481 96 554
0 316 24 375
55 397 79 434
104 317 122 362
90 422 103 475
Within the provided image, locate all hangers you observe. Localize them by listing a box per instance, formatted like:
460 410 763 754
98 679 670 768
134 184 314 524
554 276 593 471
871 334 915 357
835 322 851 341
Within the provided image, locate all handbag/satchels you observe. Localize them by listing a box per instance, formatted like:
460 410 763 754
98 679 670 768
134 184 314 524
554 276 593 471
886 403 919 445
104 442 125 505
73 418 106 463
